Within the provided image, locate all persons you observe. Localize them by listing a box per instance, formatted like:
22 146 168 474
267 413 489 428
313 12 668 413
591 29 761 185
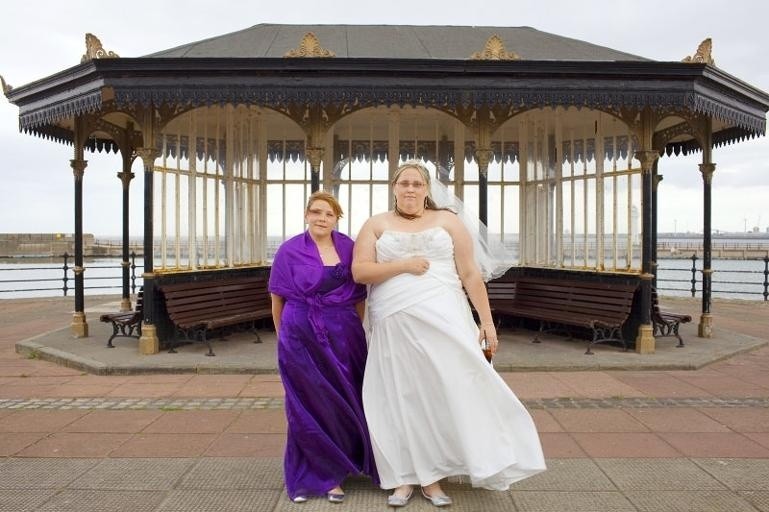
267 190 380 503
351 159 546 507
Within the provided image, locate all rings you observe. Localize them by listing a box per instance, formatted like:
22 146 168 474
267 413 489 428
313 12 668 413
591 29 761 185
495 339 500 343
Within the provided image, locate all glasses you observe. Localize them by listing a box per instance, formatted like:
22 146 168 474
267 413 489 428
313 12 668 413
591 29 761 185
396 181 425 189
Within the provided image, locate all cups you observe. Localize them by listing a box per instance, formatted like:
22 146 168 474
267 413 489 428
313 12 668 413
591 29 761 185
480 339 492 364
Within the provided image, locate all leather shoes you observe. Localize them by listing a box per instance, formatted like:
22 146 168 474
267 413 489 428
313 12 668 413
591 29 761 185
294 495 307 503
421 487 454 506
328 493 344 503
387 486 415 507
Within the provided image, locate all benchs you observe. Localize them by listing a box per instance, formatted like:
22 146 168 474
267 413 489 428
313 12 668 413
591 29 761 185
99 275 272 356
463 278 692 355
